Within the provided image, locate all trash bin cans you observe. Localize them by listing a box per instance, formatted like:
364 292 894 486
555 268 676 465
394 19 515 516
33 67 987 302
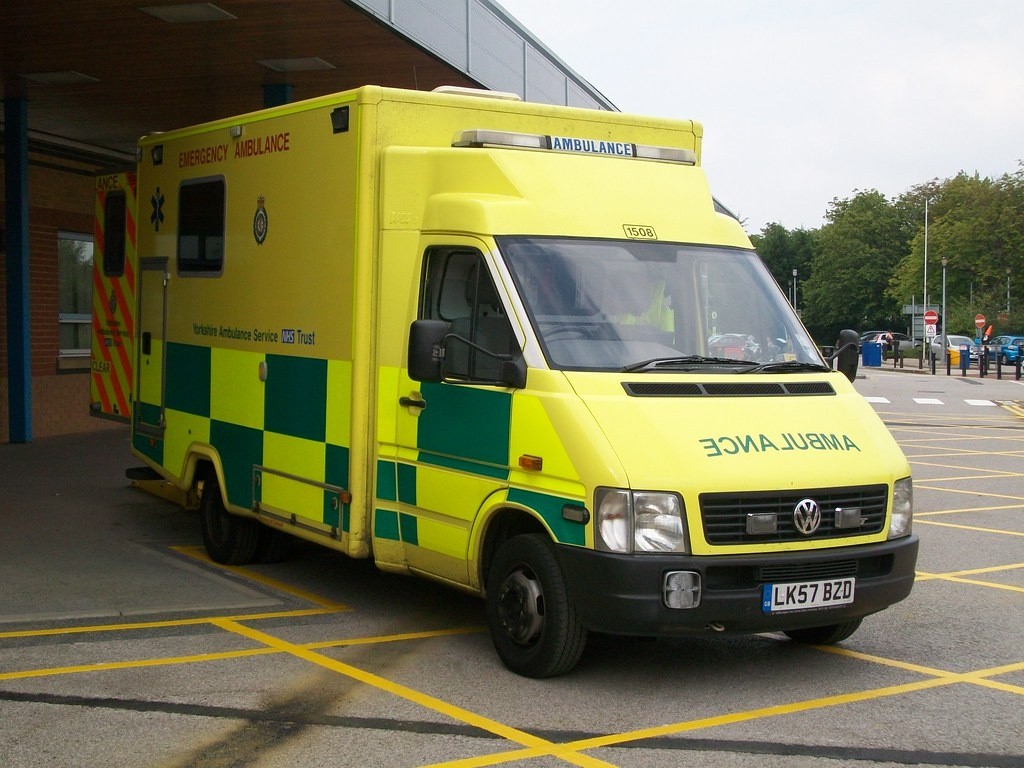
948 349 960 365
959 343 970 369
861 341 870 366
822 346 834 370
869 340 881 367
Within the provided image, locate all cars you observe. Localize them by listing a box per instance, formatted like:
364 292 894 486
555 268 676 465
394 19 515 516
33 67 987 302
931 335 979 362
706 334 755 362
859 330 927 359
981 336 1024 365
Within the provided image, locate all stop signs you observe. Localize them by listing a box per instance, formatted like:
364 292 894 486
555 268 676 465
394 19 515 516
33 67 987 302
924 310 938 324
975 314 986 327
998 315 1008 325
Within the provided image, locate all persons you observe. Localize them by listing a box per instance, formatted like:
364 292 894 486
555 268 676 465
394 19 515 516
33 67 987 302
886 329 893 351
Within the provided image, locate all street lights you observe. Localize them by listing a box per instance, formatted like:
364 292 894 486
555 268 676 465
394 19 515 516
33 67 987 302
864 314 868 331
1004 266 1012 322
787 268 798 314
940 255 948 365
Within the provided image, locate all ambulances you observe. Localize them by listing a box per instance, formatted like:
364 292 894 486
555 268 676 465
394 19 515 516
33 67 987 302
89 85 919 679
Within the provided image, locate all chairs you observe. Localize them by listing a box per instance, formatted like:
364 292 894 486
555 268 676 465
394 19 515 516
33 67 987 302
448 260 512 379
595 271 661 342
